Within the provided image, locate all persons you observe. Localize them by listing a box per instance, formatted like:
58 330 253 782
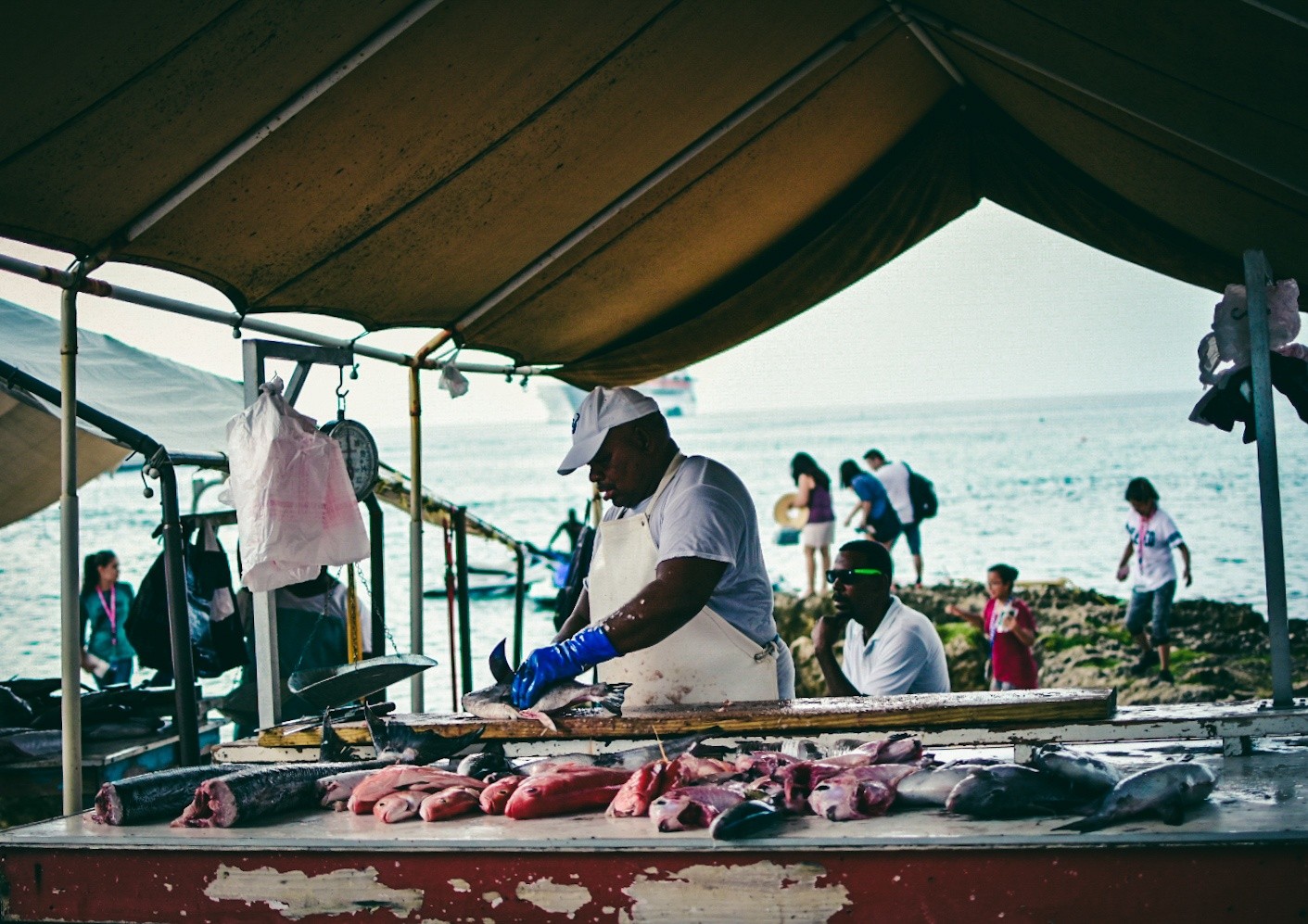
233 565 372 740
811 539 951 697
513 384 794 717
840 460 902 552
862 449 923 591
547 511 583 553
1117 477 1192 688
79 551 135 692
945 564 1038 691
789 452 835 599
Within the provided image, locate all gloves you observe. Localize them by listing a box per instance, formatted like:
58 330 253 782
510 620 617 710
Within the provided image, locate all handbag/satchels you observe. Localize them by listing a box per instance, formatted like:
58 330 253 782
124 520 245 679
903 462 938 525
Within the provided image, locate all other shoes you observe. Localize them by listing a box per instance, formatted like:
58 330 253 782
1131 652 1160 672
1150 671 1175 688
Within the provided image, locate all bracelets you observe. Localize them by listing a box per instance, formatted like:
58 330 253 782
961 612 969 620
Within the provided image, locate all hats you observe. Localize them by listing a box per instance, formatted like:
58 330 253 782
774 494 810 529
557 385 659 476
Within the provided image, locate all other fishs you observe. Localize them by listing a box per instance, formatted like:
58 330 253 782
92 676 1218 837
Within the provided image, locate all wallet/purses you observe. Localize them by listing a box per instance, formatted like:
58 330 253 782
79 655 110 681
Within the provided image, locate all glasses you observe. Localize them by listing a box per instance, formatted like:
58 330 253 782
826 570 883 584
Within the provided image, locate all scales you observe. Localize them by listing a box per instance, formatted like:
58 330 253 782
289 409 438 711
